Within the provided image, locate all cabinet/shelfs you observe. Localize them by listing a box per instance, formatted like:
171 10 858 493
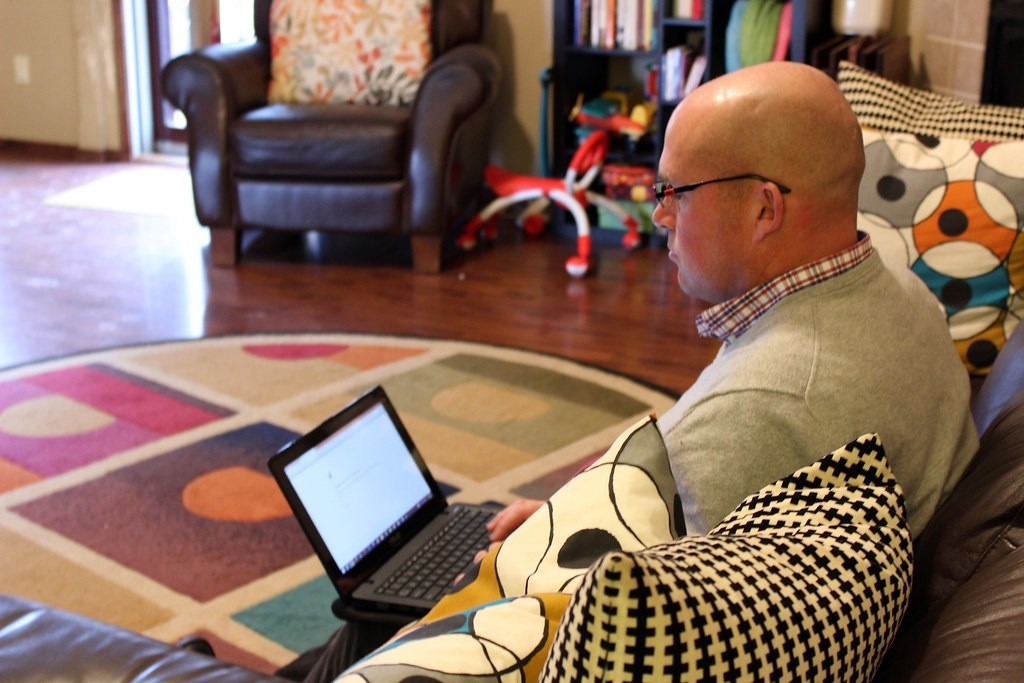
540 0 830 255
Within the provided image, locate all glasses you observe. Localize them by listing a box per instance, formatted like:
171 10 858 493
653 173 791 208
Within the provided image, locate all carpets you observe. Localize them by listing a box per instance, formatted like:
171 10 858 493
0 327 683 675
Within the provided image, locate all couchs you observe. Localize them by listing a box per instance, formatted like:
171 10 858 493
159 0 502 274
0 319 1024 683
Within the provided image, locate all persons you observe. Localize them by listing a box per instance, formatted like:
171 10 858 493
179 61 979 683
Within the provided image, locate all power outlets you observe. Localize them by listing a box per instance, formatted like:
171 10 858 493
12 53 34 86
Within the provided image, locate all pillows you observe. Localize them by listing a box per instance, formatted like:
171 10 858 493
834 60 1024 403
539 433 916 683
264 0 433 108
331 411 688 682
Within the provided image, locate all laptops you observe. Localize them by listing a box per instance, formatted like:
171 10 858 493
265 381 527 618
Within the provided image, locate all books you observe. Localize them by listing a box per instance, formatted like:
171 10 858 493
572 0 707 99
